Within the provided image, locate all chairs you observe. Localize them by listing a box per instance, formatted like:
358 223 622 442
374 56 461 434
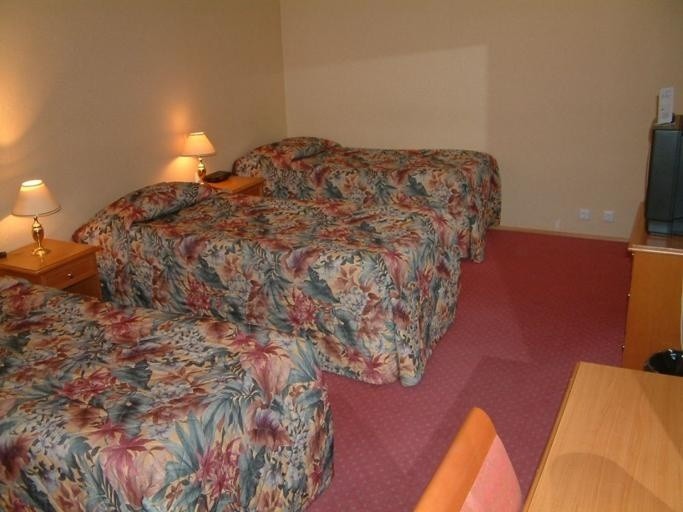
411 408 523 512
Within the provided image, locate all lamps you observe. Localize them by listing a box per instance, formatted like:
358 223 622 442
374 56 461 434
11 180 62 256
181 131 218 186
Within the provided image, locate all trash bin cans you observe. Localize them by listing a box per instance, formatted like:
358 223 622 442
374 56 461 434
647 351 683 378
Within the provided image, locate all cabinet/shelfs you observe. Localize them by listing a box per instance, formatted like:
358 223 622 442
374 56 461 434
620 199 682 371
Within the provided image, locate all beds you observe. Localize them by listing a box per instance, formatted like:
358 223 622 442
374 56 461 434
0 275 334 512
233 136 502 264
72 181 460 386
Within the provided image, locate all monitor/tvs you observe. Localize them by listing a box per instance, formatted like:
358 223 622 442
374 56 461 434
645 115 683 237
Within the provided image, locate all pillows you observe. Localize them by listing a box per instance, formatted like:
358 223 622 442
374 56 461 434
256 137 332 162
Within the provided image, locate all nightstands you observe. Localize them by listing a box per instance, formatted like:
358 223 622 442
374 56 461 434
1 239 105 300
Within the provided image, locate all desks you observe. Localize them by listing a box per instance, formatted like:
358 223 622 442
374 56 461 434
521 359 683 512
